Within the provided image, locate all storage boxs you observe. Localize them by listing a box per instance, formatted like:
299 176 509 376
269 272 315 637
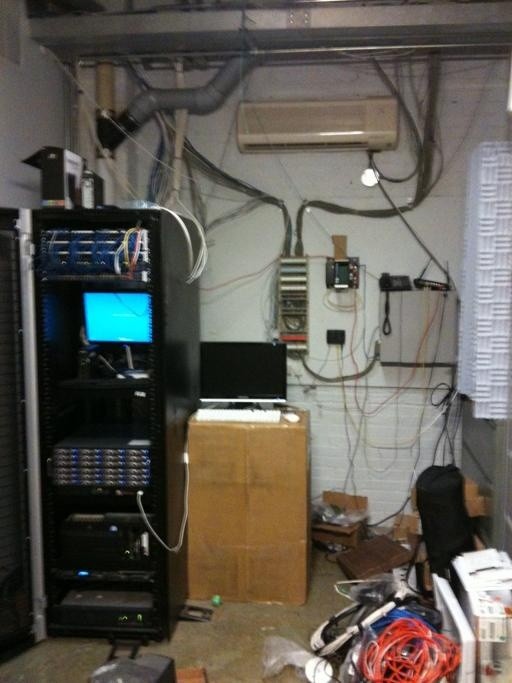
311 489 369 549
393 472 489 591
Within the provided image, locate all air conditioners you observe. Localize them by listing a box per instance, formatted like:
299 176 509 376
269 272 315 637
234 97 400 155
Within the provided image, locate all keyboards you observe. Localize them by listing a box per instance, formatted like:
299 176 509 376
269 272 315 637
196 408 281 424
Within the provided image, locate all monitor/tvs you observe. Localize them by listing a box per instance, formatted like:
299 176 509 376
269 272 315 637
83 292 154 345
200 341 287 408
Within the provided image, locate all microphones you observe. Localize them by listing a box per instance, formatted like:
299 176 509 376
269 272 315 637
414 278 449 290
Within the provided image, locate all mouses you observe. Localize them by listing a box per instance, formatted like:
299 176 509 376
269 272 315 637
284 412 300 422
